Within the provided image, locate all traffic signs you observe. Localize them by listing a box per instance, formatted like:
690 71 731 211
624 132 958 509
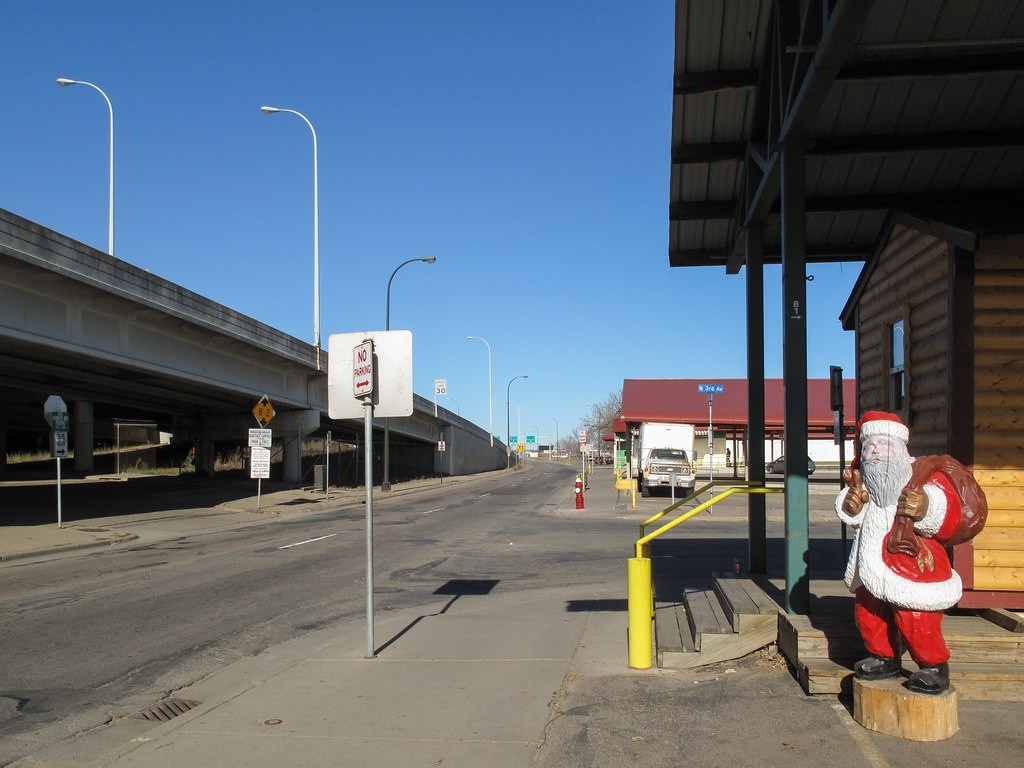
54 430 68 458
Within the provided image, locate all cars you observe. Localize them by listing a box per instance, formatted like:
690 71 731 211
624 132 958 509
767 455 816 475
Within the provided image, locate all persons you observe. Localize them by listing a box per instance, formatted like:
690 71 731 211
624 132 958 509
726 448 731 466
834 410 963 694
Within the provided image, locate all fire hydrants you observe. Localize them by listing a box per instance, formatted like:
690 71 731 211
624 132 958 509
573 478 585 509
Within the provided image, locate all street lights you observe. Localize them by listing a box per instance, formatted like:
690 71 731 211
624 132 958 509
507 375 528 451
468 336 494 448
57 77 115 257
586 403 600 465
259 104 322 349
546 417 559 461
385 256 437 328
528 426 538 459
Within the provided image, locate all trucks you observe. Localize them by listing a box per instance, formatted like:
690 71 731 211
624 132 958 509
636 421 698 498
